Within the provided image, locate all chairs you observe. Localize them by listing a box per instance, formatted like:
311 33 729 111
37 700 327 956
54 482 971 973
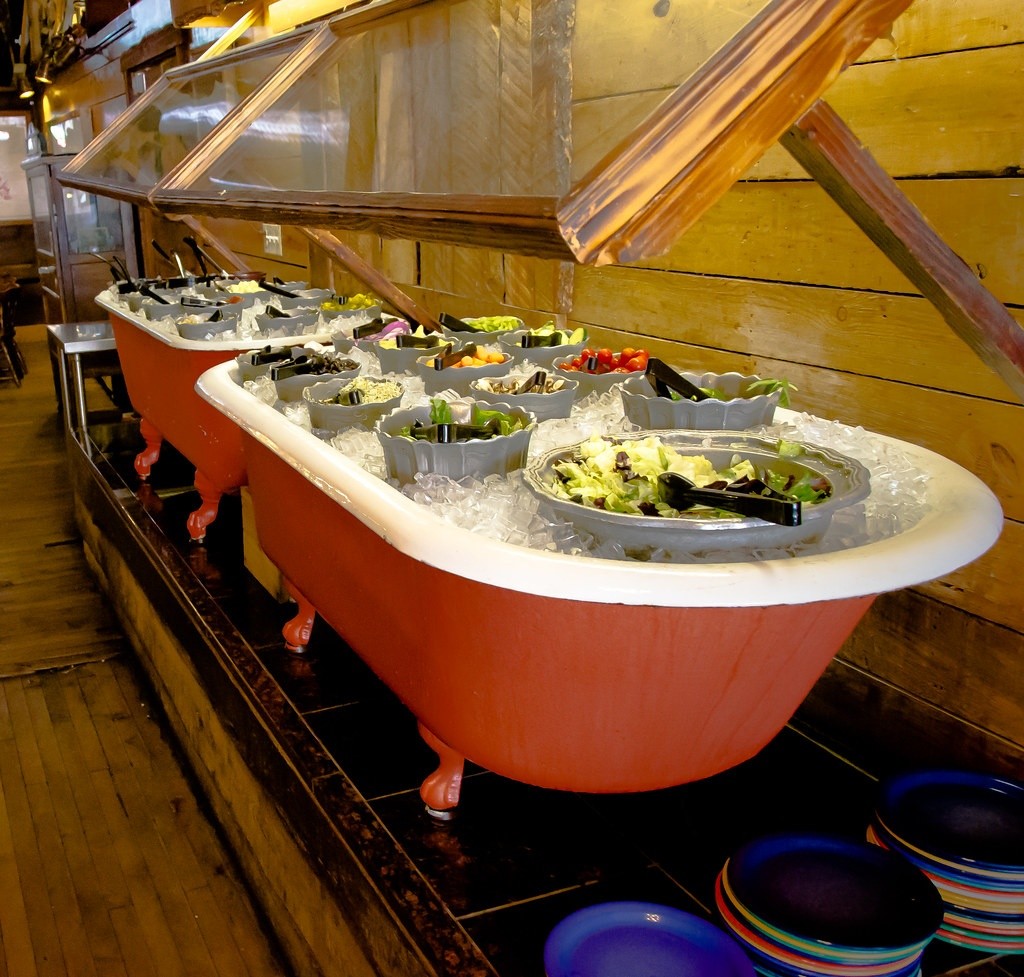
0 275 29 389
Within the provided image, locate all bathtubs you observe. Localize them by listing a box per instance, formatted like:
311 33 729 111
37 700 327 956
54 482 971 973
191 313 1006 825
90 277 408 544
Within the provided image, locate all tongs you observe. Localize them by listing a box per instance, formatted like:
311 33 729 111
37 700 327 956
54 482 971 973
396 335 439 349
515 372 546 396
274 277 286 286
434 343 477 371
439 312 478 333
645 358 710 403
332 294 348 305
410 419 501 444
658 472 801 526
181 297 226 307
259 280 301 297
208 309 223 322
136 284 169 304
334 389 363 406
353 318 399 339
266 305 291 319
252 345 292 366
522 331 562 348
271 355 323 382
578 358 600 375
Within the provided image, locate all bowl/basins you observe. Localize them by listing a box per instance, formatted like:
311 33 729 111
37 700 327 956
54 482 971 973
117 271 870 554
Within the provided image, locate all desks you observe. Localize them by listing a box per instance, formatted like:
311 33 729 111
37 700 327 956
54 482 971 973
46 320 117 458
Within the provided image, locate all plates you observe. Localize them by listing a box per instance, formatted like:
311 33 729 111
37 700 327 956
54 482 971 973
544 770 1024 977
